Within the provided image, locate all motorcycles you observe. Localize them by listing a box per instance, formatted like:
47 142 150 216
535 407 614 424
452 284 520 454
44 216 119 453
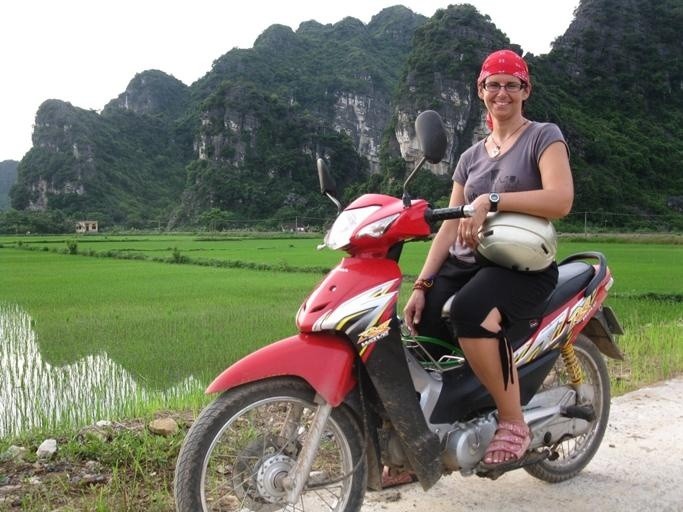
172 110 625 512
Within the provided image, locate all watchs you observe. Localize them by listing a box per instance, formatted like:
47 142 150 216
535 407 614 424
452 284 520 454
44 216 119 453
489 192 500 212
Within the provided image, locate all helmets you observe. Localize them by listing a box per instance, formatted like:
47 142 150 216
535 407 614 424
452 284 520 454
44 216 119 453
474 211 557 271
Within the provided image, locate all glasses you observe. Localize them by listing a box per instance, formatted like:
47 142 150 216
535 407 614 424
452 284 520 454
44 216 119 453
482 81 527 92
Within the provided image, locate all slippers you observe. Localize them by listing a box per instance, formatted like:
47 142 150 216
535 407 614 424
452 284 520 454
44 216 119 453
380 467 414 489
480 424 532 470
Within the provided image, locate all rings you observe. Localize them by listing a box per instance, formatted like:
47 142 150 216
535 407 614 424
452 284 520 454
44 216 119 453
465 231 472 237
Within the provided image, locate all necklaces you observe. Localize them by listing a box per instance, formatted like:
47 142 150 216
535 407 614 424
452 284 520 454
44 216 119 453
489 119 529 157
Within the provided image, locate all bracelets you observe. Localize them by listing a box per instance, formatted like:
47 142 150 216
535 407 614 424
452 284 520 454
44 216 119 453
412 279 433 291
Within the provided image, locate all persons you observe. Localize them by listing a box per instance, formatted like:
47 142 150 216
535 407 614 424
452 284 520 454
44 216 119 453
366 50 575 491
